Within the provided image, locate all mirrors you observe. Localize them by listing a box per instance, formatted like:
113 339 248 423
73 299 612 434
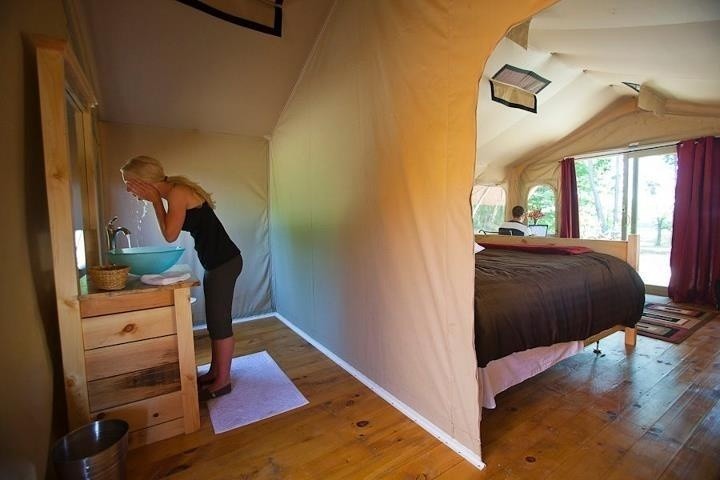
33 34 102 282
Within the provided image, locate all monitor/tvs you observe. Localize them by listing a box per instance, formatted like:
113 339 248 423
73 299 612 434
528 225 548 236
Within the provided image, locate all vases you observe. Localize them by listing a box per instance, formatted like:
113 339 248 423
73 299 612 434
532 218 538 225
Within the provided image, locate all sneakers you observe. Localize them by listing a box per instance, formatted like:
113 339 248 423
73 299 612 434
198 383 231 401
198 375 215 386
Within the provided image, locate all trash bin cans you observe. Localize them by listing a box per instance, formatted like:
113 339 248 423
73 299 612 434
52 418 129 480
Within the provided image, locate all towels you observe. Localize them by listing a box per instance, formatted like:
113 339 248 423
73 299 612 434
138 271 193 286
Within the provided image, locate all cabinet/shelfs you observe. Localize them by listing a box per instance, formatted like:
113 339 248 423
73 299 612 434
54 267 203 455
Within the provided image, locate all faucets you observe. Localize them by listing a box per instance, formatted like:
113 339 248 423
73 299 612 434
105 216 130 249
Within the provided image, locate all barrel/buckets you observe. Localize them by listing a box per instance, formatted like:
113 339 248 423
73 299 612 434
51 418 129 480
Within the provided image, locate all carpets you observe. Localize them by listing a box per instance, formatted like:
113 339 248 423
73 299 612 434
195 348 310 436
631 296 720 344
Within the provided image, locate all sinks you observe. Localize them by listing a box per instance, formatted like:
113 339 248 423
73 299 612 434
105 246 185 276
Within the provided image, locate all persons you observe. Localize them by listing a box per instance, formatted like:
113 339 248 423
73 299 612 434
118 153 244 403
500 203 531 238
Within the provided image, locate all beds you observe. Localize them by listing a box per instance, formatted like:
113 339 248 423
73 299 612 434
475 230 647 416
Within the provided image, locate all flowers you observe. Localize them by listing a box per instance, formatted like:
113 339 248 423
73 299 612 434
526 208 546 221
